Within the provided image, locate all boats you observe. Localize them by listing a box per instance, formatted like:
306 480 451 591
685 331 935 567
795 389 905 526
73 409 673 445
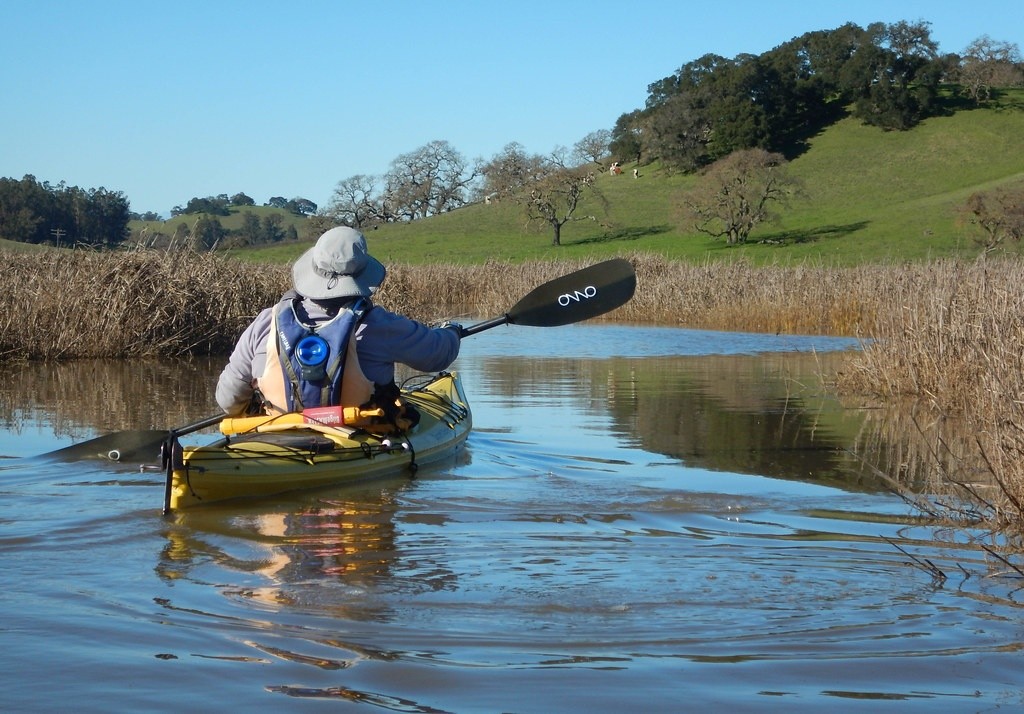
163 371 471 513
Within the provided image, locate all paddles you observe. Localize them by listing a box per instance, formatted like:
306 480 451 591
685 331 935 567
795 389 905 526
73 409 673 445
36 260 640 491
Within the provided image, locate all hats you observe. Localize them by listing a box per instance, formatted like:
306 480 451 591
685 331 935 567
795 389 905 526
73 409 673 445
291 226 387 299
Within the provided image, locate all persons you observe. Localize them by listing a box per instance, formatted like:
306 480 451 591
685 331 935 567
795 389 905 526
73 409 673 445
215 226 463 424
